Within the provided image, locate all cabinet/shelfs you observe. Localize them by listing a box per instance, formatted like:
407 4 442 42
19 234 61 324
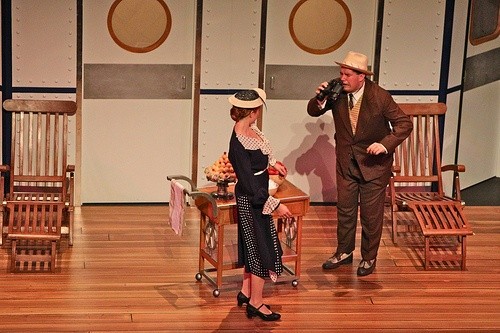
167 174 310 296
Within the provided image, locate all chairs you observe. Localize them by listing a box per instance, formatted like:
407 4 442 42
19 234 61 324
0 100 78 273
385 102 474 273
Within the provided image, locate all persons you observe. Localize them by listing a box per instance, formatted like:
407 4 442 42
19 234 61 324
228 87 292 321
307 51 413 276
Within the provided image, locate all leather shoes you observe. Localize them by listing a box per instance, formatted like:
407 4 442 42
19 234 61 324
358 257 376 275
322 252 353 269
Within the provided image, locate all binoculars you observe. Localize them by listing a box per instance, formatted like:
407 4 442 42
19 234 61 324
317 78 343 100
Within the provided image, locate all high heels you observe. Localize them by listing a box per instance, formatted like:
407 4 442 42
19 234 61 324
246 303 281 321
237 292 271 309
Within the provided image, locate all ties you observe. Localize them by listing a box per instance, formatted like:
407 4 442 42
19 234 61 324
349 93 354 109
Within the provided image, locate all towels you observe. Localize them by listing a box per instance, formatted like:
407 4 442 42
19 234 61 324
166 178 186 238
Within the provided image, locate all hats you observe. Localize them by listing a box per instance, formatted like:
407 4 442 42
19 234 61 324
334 52 374 76
227 87 267 108
